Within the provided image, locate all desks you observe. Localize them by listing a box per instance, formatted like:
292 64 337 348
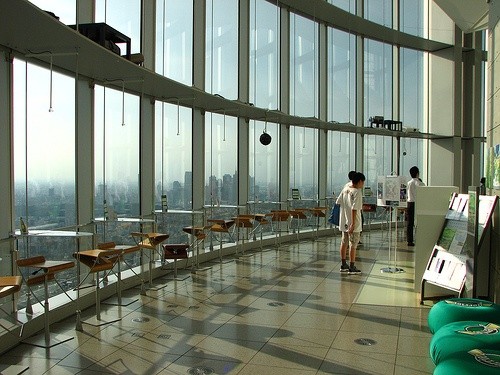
204 197 399 262
153 210 204 274
94 218 157 295
9 229 93 331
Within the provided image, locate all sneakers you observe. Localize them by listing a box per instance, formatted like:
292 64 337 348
357 241 363 246
340 265 349 272
348 266 361 274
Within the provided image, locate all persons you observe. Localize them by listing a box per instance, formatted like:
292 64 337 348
335 174 364 275
328 170 365 247
406 166 425 247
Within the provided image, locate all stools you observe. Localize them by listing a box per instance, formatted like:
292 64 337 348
131 231 190 291
16 256 76 348
0 276 24 337
74 242 141 326
183 204 408 271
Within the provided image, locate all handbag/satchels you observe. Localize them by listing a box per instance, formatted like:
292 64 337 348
328 203 340 226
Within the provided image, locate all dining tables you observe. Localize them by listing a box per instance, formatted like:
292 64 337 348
383 120 402 131
65 23 131 62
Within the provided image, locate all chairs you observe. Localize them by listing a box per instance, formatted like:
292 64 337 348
372 116 384 128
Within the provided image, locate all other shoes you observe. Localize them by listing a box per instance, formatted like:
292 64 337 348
407 242 415 246
